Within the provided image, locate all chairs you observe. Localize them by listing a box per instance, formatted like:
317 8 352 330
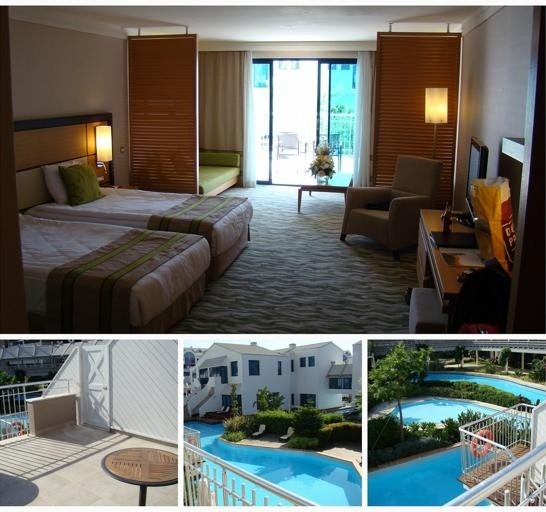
339 153 443 259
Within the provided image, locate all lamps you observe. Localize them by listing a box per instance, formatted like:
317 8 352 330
424 86 448 160
95 126 113 172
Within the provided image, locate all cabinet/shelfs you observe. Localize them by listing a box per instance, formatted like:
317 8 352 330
415 208 484 312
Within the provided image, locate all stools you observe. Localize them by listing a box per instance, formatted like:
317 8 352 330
409 289 448 333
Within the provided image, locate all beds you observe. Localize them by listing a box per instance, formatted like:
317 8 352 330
25 187 253 284
19 212 212 333
199 151 242 197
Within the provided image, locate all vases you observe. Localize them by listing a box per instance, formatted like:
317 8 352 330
316 176 328 186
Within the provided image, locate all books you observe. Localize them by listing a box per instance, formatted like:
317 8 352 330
441 250 486 268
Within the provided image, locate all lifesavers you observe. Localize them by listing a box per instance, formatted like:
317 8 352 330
470 429 494 458
8 422 24 439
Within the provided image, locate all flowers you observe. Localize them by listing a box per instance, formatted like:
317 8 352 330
100 447 178 506
304 142 335 179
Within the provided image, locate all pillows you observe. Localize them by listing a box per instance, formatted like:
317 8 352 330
58 161 106 208
41 159 78 203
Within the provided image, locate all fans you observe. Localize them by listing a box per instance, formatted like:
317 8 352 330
297 174 353 214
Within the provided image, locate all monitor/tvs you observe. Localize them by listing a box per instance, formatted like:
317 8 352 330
451 135 489 228
